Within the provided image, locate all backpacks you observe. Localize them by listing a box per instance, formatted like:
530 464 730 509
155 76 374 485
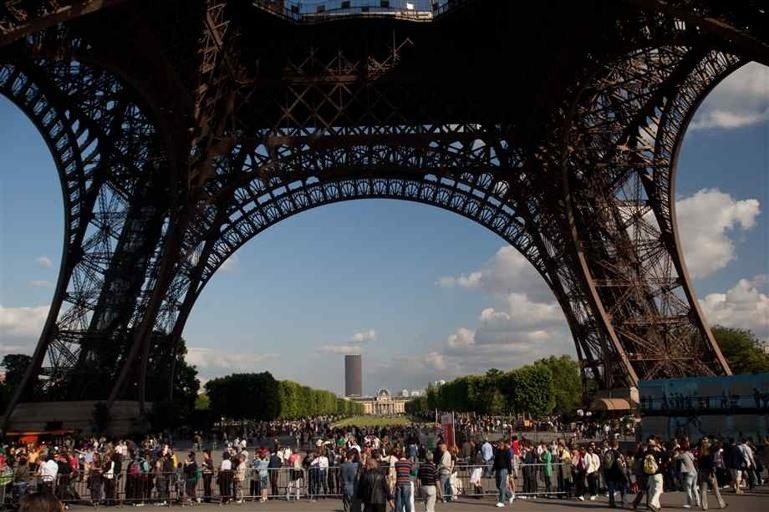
643 454 659 475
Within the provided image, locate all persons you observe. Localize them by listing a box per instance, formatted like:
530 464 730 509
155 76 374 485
1 432 202 512
569 414 638 507
292 412 361 500
452 414 572 507
191 418 302 504
629 432 769 512
340 422 458 512
